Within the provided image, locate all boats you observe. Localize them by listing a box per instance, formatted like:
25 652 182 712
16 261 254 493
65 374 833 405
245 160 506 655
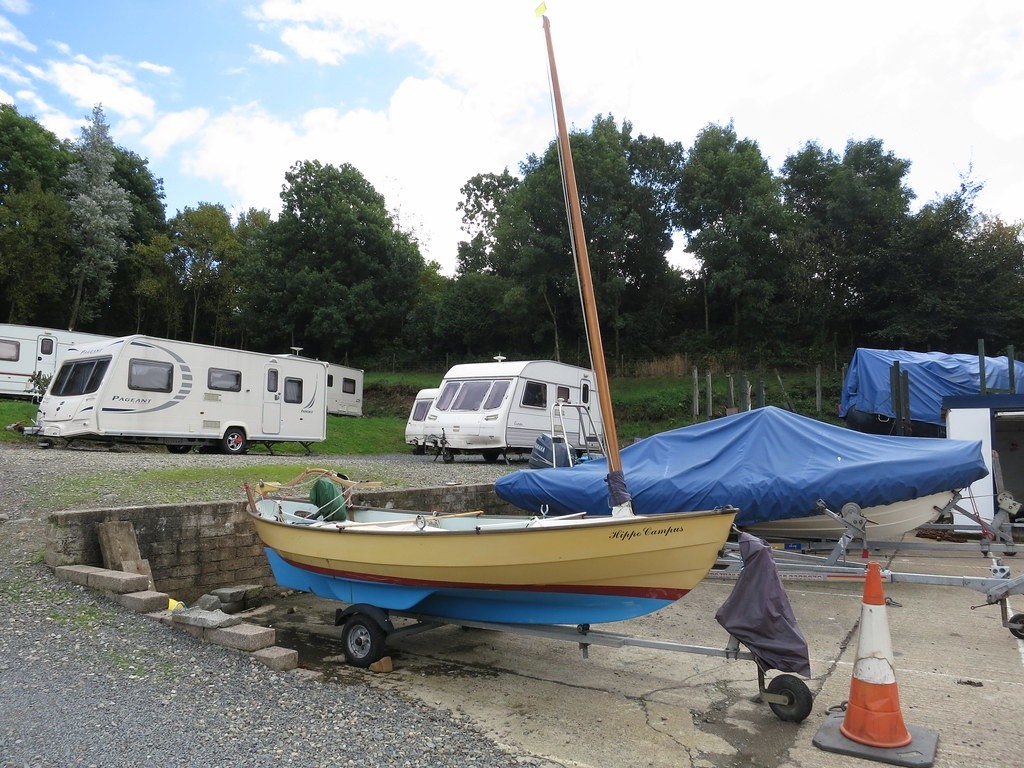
490 404 989 547
242 10 738 630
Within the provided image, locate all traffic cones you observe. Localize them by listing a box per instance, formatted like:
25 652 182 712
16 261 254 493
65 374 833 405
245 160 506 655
839 561 912 747
